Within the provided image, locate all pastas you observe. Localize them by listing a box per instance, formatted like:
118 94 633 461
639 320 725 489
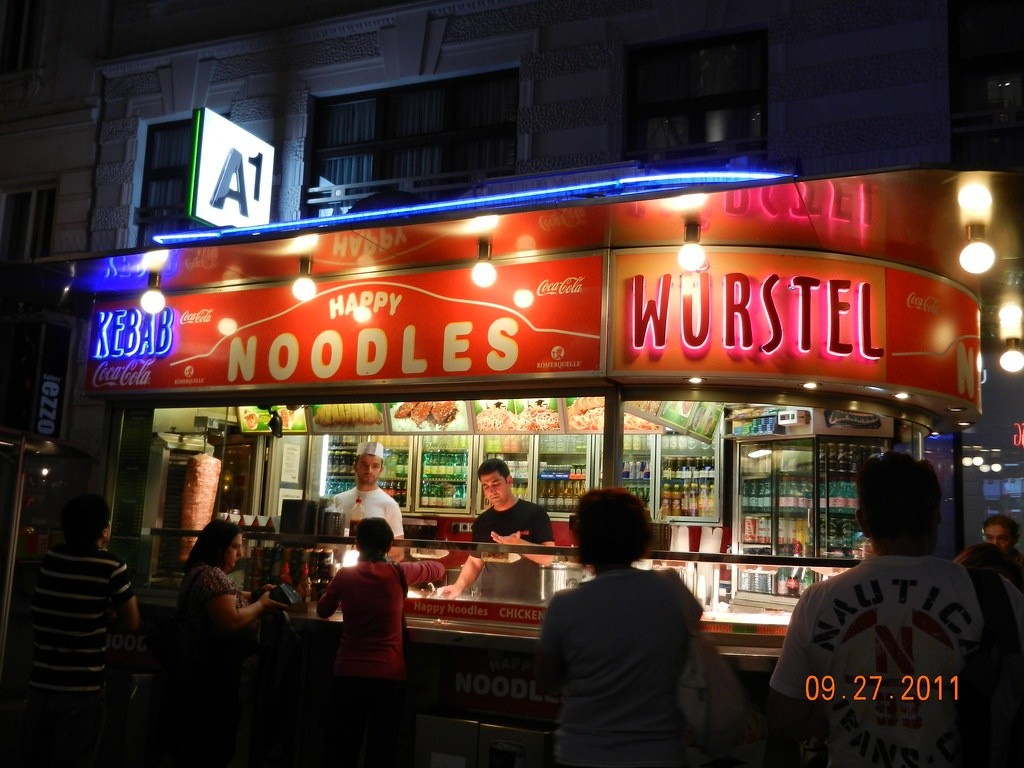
474 400 659 432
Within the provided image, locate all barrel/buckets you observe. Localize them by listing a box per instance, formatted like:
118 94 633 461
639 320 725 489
280 499 319 536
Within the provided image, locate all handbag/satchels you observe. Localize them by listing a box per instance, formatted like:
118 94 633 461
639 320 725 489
665 581 753 756
952 564 1024 768
142 608 191 681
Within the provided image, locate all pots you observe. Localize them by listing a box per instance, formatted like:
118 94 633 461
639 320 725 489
530 562 582 602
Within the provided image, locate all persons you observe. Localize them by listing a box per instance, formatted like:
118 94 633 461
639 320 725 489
952 513 1024 594
21 493 140 768
333 441 405 561
769 450 1024 768
159 520 288 768
317 517 446 768
441 457 555 604
531 486 704 768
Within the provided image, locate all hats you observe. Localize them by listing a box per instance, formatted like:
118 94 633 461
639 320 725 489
356 442 384 461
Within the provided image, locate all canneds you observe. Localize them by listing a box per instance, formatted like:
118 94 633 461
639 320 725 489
742 516 812 545
817 442 882 474
242 546 335 602
660 456 715 473
817 518 866 548
622 460 650 480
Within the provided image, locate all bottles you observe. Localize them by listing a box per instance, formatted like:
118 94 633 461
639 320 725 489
660 454 715 519
326 442 409 509
820 475 859 515
741 476 814 599
245 547 334 604
537 462 586 512
482 454 528 510
419 449 469 510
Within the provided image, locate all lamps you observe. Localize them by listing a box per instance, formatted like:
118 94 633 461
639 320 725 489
291 255 319 305
258 405 283 439
139 272 166 315
470 242 498 290
958 223 1024 376
674 222 709 272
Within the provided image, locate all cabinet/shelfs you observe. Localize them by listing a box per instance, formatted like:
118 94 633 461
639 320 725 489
327 435 720 524
